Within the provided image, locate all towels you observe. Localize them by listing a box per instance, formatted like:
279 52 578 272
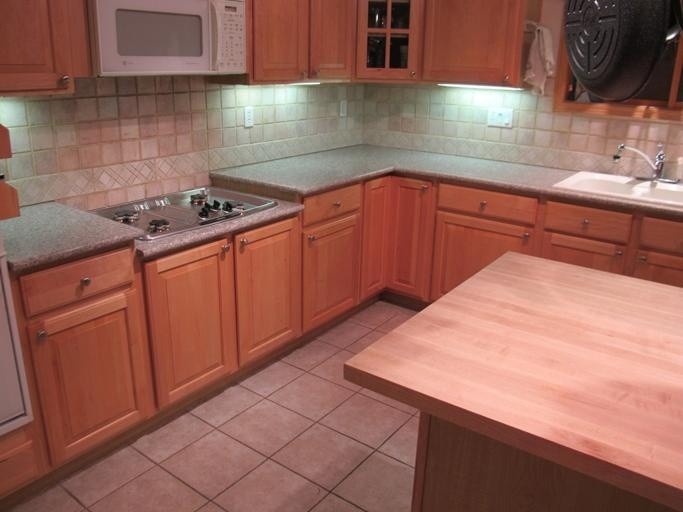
522 22 557 97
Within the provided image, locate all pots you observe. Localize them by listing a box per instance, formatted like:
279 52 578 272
561 1 673 101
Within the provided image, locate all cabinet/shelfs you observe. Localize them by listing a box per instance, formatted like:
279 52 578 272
299 175 539 331
134 217 299 421
542 196 683 287
0 244 156 510
0 0 76 99
358 0 543 87
554 0 682 127
205 1 354 85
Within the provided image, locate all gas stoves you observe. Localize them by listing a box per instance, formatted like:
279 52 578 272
104 194 262 237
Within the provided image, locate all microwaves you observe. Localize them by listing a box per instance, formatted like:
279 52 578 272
87 1 247 77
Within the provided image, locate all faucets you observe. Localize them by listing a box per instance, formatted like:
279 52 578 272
613 142 666 180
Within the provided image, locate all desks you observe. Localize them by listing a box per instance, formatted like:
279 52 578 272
342 248 683 512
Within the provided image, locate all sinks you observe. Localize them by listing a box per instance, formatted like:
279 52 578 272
553 169 683 206
